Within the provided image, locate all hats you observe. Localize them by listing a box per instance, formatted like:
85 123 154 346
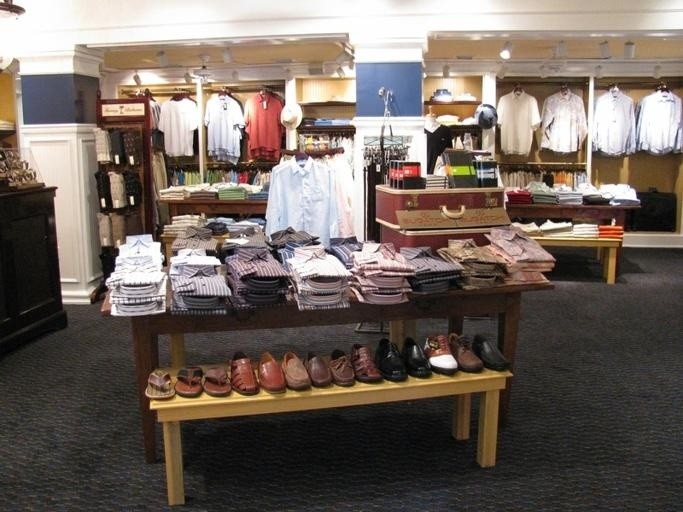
475 104 498 128
280 103 302 129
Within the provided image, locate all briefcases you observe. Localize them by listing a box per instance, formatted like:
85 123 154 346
627 191 676 231
375 184 504 228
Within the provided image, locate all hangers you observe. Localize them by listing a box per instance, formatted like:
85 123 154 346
135 83 282 102
172 146 346 176
509 80 671 96
502 161 589 176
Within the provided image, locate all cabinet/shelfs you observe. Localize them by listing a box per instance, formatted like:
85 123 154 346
424 100 483 136
296 99 357 131
1 184 70 362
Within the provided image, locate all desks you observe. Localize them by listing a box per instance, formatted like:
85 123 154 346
148 355 515 508
157 199 268 225
102 272 556 466
527 238 622 285
503 202 642 285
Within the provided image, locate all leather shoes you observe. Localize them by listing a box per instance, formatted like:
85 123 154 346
230 333 509 395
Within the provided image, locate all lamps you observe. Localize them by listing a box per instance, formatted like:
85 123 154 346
501 39 514 61
599 38 637 61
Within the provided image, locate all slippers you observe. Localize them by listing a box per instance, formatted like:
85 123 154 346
146 366 231 398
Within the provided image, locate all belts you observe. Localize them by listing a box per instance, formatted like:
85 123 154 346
364 147 408 242
